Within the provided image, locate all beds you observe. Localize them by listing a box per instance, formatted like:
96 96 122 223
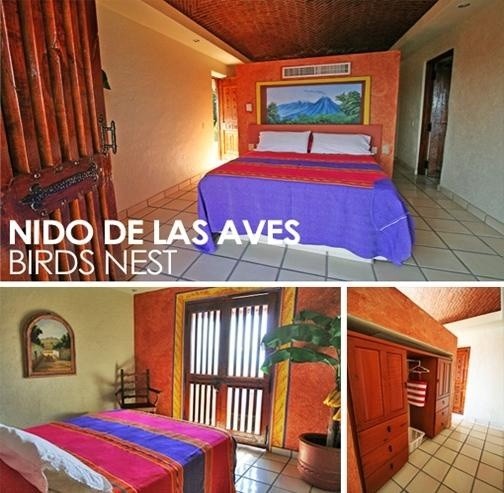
198 123 399 264
0 407 235 492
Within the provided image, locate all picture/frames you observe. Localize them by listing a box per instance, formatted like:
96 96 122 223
255 74 370 125
19 311 76 378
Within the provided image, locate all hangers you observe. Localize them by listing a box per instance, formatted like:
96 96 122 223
409 360 430 373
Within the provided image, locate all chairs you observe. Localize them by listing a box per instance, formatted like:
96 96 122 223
114 367 161 413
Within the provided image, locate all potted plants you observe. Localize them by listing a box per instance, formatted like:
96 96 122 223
258 309 340 493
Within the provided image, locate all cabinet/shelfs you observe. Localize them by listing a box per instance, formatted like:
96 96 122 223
346 335 409 493
409 356 452 439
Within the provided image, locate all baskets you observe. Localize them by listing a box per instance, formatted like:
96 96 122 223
409 426 425 453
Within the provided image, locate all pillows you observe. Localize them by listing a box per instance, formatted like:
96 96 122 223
0 422 112 492
252 129 375 155
